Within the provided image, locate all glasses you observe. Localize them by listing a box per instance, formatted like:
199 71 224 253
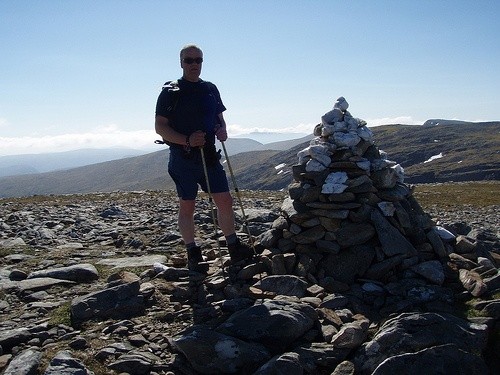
182 58 202 64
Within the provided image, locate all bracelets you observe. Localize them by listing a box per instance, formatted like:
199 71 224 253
186 136 190 149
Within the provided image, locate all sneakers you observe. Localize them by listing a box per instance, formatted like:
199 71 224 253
187 246 209 271
226 238 258 266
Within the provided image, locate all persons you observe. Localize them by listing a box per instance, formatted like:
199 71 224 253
155 46 254 267
